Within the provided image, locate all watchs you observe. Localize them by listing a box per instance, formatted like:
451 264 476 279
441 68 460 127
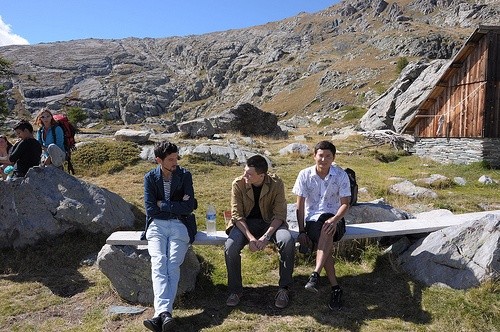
299 230 306 234
264 235 270 239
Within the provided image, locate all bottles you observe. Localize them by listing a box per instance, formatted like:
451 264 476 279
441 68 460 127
299 234 308 254
206 206 216 239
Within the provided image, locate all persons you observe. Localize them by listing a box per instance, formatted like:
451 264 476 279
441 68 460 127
0 120 42 180
0 134 13 174
291 140 352 310
225 155 295 308
34 110 66 172
140 140 198 332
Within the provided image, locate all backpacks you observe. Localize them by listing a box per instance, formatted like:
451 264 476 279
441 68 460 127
345 167 358 206
39 114 76 161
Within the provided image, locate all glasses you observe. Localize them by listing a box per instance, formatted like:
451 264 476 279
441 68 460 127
40 114 51 118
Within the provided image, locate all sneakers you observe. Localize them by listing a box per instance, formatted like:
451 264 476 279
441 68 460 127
328 288 343 312
226 291 243 305
304 272 319 294
143 312 175 332
274 287 289 308
